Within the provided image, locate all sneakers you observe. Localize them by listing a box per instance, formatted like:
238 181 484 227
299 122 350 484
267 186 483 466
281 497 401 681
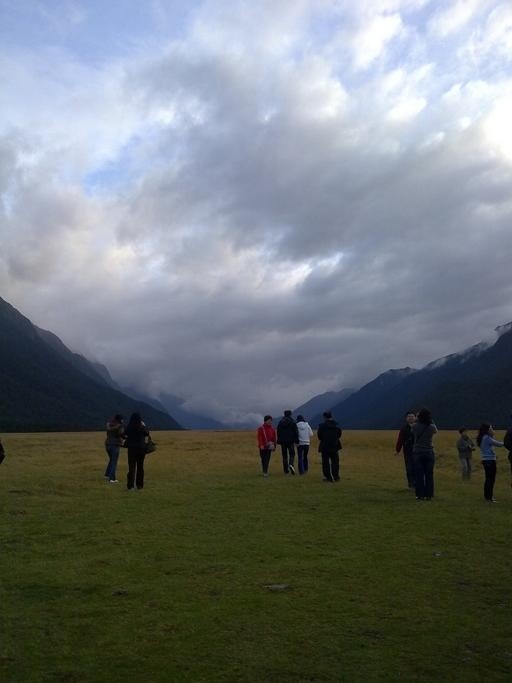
323 476 341 484
284 464 295 475
104 474 119 483
487 496 498 503
261 471 271 478
415 495 432 501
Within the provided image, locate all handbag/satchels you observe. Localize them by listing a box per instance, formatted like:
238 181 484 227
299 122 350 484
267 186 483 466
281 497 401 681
266 441 275 451
145 434 156 454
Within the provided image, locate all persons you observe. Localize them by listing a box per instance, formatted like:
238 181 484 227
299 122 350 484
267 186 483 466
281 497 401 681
457 427 476 479
125 414 150 489
477 424 505 503
296 415 313 474
256 415 277 477
503 414 512 464
317 413 343 483
0 440 5 464
104 415 125 483
276 410 299 476
393 411 422 489
411 408 438 503
